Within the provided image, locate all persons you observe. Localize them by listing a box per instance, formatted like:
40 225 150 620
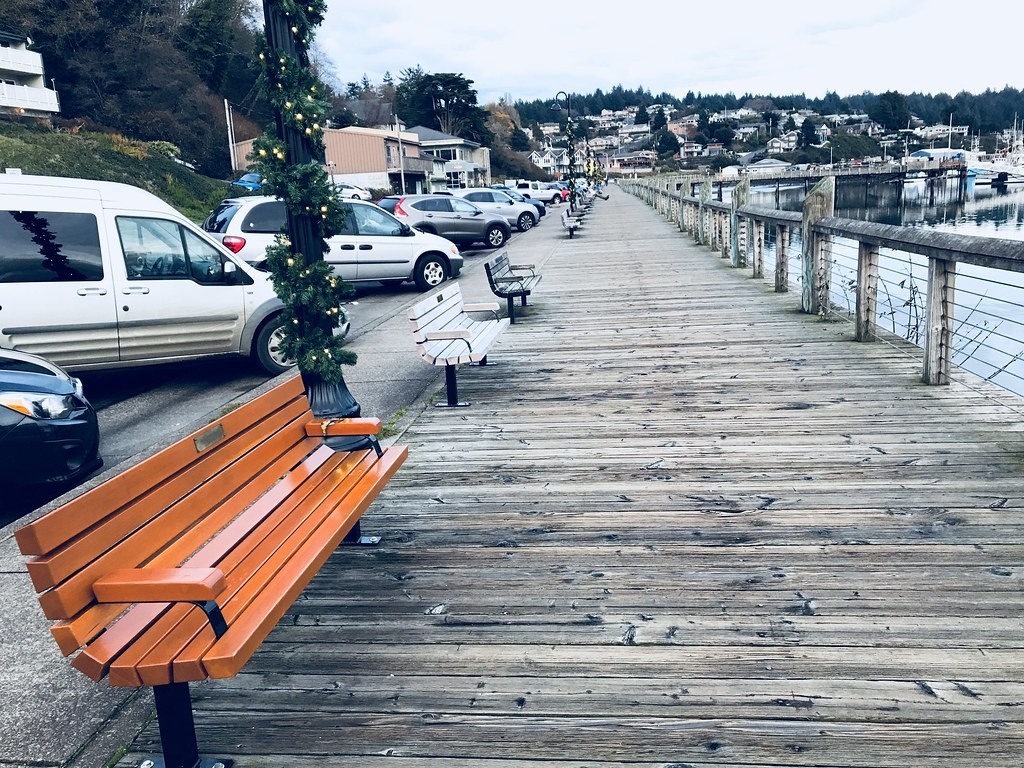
568 183 576 212
587 176 608 194
588 184 609 200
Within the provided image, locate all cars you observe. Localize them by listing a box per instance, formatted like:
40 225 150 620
0 344 105 530
328 182 373 202
480 177 590 218
229 172 265 193
200 195 465 291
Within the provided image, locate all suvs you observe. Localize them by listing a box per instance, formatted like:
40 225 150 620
365 193 512 249
432 187 540 233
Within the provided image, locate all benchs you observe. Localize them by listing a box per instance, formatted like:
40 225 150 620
14 374 409 768
560 191 595 238
484 251 542 324
408 282 511 407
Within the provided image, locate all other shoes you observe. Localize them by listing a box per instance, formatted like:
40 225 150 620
605 195 609 200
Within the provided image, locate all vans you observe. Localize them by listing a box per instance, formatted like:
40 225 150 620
0 172 307 376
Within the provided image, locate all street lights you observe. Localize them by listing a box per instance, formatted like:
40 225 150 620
550 91 576 204
